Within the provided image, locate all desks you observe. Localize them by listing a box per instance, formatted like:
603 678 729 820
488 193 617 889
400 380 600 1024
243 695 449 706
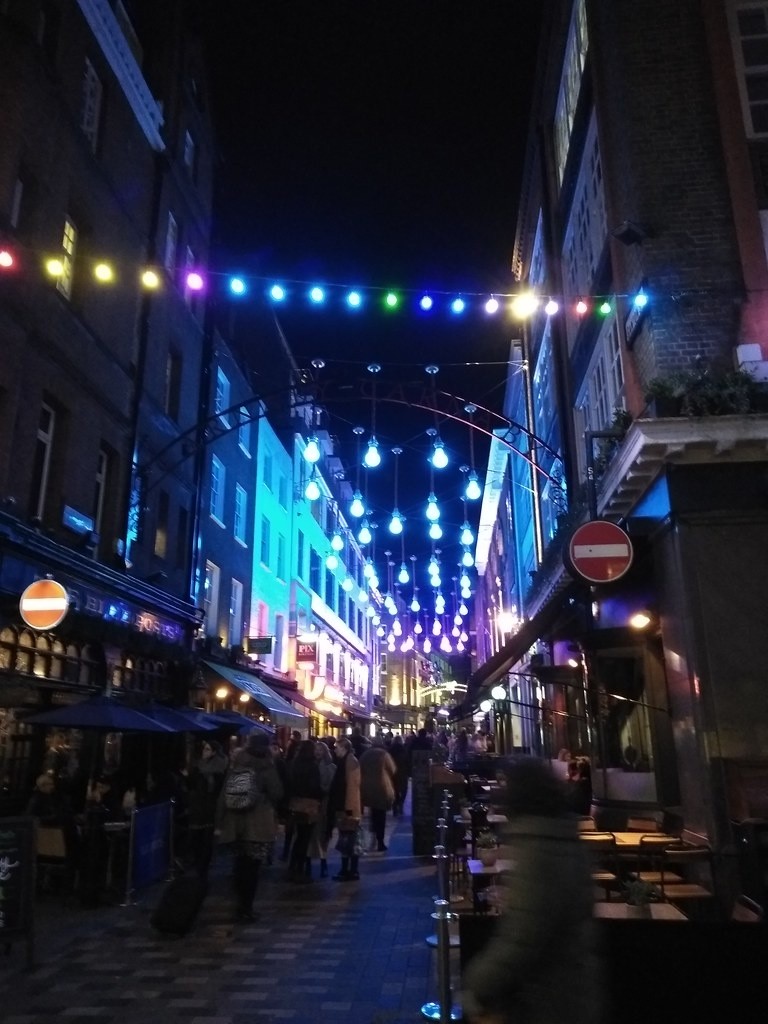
595 902 688 920
578 831 682 853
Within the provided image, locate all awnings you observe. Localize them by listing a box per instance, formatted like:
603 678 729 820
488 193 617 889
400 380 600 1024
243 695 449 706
269 684 353 723
203 660 304 719
447 623 586 721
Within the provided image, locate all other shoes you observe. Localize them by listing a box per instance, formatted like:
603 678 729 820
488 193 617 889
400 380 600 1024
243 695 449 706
378 839 388 851
320 868 328 879
332 869 360 882
237 912 261 923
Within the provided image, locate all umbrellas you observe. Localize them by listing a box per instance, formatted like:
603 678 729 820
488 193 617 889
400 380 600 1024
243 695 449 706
135 697 277 736
23 696 177 793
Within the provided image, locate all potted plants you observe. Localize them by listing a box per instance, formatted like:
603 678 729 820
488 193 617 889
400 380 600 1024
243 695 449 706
581 409 635 477
475 831 499 867
622 879 660 918
467 802 490 821
641 361 768 421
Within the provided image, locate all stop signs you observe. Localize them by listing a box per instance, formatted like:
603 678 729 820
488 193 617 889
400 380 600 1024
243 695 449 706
571 521 635 585
19 579 72 631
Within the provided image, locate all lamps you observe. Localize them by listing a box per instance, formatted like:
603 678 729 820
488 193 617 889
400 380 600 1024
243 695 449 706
71 530 102 558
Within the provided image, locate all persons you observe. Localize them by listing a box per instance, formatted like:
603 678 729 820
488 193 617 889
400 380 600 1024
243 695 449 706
26 774 54 816
454 756 596 1024
558 748 592 815
187 727 413 920
410 728 495 771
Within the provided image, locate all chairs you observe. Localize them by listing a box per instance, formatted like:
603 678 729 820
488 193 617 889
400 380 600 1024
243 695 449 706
577 816 714 902
466 859 519 913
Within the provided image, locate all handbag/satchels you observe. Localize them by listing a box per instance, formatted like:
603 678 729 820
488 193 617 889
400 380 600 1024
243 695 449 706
337 816 365 857
286 797 321 825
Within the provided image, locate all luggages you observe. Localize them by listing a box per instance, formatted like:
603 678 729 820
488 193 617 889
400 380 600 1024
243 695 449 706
150 872 212 939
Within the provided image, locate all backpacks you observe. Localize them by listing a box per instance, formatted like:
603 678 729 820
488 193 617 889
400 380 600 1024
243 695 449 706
223 767 259 812
